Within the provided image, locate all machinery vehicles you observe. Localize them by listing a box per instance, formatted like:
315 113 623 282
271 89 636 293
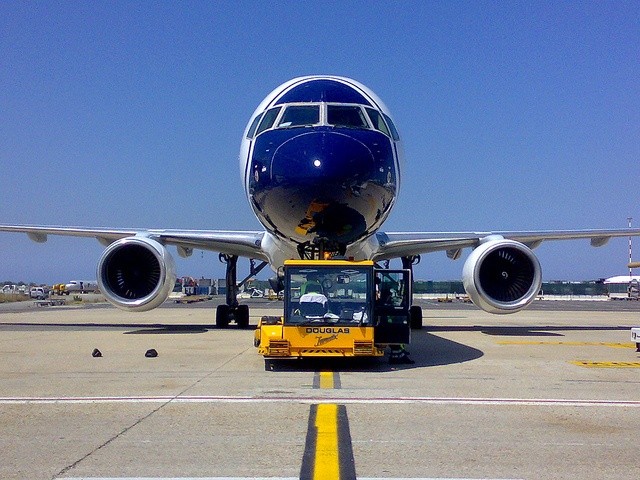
254 258 414 369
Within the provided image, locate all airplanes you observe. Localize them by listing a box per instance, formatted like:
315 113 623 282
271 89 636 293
64 278 97 295
0 73 640 328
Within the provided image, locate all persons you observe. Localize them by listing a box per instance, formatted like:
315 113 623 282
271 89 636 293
300 272 324 296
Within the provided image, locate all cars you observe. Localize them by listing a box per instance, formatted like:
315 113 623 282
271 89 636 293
1 284 49 300
